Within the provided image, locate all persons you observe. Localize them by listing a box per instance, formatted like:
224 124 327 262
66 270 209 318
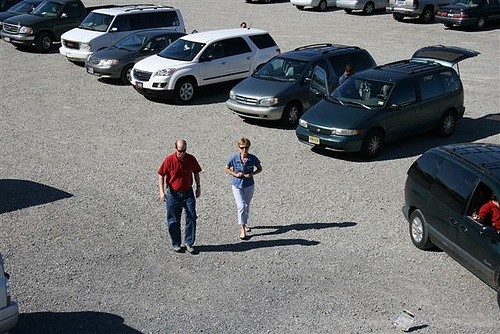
339 64 357 98
157 139 202 253
225 138 262 238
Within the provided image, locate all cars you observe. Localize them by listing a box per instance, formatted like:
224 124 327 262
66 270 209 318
434 0 500 32
245 0 289 4
57 3 186 66
84 27 190 85
0 0 43 31
290 0 336 11
334 0 389 16
0 250 21 334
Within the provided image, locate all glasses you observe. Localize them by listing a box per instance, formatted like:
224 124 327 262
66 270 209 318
238 146 249 149
176 148 186 152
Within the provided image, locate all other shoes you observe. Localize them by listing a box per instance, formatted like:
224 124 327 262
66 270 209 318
239 225 250 240
172 245 181 251
185 243 194 252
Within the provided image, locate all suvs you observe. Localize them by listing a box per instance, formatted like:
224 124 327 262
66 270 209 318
294 43 482 162
401 141 500 309
128 21 282 104
224 42 378 128
0 0 123 54
385 0 453 24
